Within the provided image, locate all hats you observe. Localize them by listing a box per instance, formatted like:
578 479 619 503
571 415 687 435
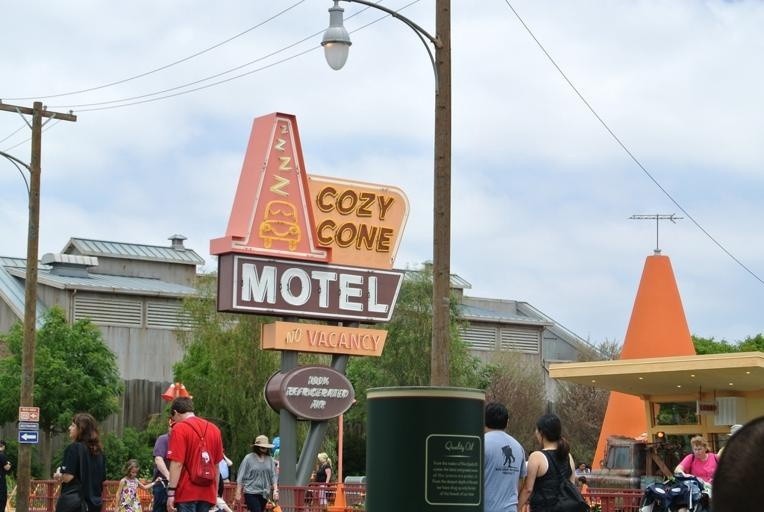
250 435 275 449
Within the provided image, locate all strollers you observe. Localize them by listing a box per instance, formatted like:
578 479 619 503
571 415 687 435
641 476 711 511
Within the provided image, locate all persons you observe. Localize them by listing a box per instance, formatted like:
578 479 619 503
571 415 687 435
484 403 594 512
0 440 11 512
674 415 764 512
53 397 332 512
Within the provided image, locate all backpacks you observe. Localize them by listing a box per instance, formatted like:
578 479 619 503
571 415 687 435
538 450 591 511
180 419 216 486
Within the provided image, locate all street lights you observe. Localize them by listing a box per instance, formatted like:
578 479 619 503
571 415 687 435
318 1 449 386
